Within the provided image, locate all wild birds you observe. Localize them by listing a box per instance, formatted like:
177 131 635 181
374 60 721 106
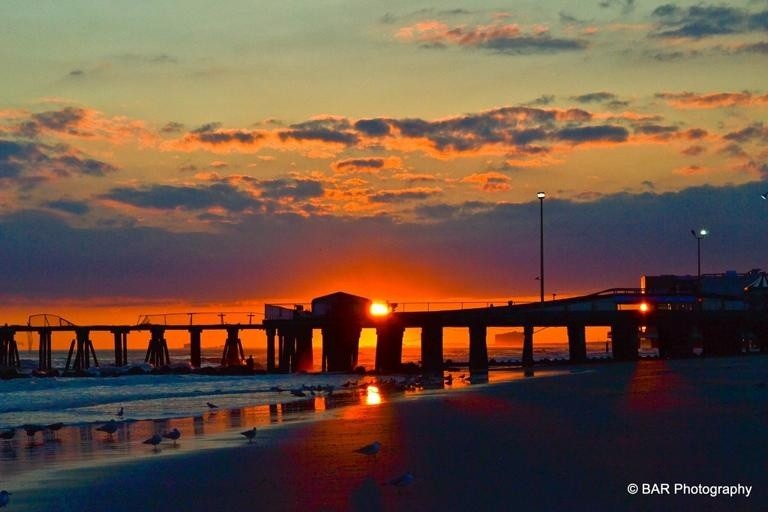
380 468 416 495
353 441 381 462
0 490 12 508
0 428 16 439
240 427 256 443
207 402 218 410
141 428 180 449
269 370 469 398
96 419 118 434
114 407 123 416
47 422 63 431
27 424 35 436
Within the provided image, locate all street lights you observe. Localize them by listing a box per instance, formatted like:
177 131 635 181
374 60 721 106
537 191 546 303
691 229 707 280
